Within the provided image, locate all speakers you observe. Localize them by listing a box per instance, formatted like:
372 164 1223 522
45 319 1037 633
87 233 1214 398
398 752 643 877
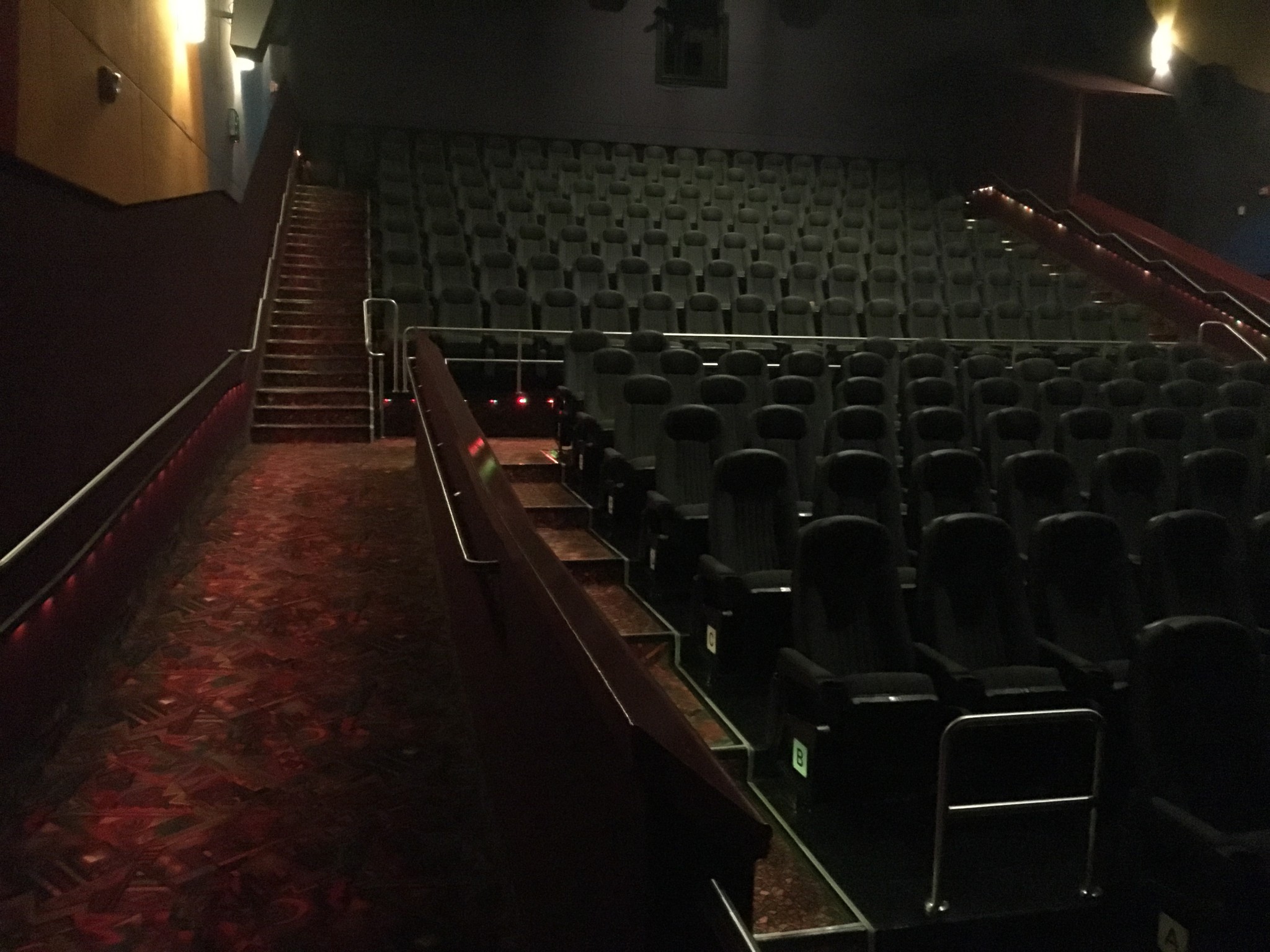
231 0 279 63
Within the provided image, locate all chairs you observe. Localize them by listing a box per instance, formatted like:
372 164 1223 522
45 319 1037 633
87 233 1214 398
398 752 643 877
363 124 1270 910
